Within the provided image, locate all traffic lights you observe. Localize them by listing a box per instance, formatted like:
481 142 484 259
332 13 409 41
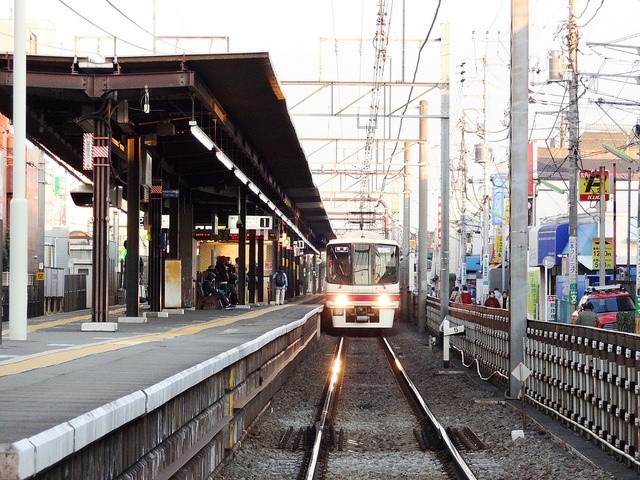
526 197 535 227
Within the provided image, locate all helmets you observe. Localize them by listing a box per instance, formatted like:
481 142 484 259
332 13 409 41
584 302 593 310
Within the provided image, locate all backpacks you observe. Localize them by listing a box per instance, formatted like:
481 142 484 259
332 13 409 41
276 271 286 287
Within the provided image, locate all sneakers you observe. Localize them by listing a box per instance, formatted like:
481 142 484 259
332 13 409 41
228 304 236 307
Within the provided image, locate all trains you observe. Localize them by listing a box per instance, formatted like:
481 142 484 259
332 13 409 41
323 229 402 329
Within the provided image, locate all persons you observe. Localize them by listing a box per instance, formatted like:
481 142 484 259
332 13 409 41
272 265 288 305
575 301 597 327
484 291 501 308
450 284 459 303
498 291 509 309
430 287 438 298
461 286 471 304
493 288 502 300
455 292 461 303
201 256 265 309
123 240 143 314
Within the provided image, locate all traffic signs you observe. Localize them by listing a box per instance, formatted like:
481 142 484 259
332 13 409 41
591 236 615 270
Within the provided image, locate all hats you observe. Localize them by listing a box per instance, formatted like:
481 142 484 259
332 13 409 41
279 266 284 270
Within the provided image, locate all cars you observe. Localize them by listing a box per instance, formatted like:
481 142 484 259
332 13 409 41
570 285 638 333
